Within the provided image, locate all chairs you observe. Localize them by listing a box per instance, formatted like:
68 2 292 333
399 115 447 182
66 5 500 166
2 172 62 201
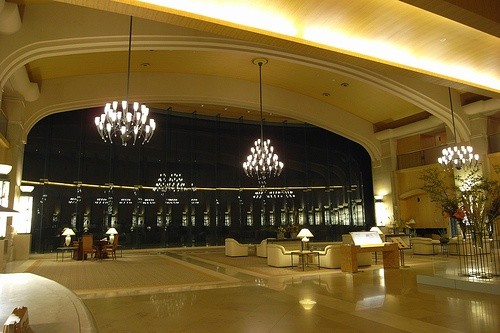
73 234 123 261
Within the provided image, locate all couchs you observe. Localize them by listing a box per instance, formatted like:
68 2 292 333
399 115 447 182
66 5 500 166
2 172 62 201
225 238 248 257
256 239 371 268
410 234 494 255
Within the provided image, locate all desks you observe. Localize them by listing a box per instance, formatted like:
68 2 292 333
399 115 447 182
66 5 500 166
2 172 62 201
384 234 411 249
266 238 304 252
0 272 96 333
291 251 320 271
340 242 399 273
56 247 78 262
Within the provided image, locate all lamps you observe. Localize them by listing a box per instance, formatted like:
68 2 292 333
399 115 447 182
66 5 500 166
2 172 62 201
369 227 383 236
0 164 35 241
374 195 383 203
438 87 479 171
61 228 75 245
409 219 416 224
297 227 314 251
242 58 284 179
95 15 156 146
106 227 118 244
